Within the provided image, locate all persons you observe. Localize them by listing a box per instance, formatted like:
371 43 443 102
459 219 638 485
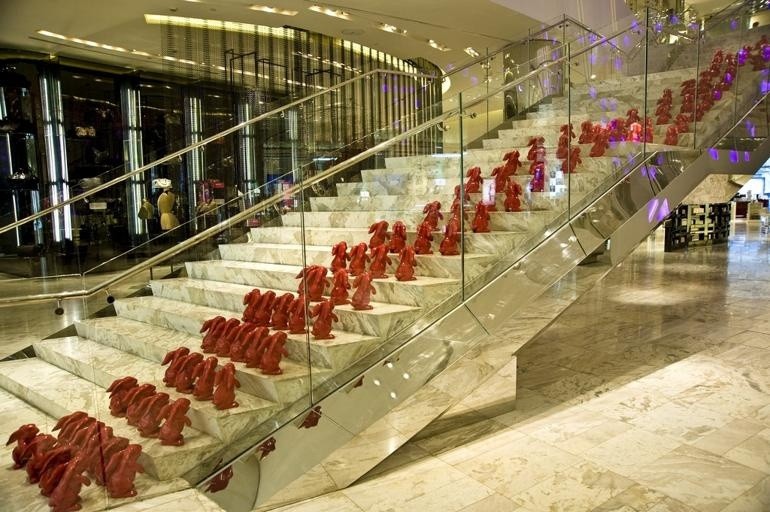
138 198 156 220
157 188 181 230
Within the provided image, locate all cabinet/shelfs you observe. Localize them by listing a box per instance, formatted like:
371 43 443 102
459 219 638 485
665 201 732 251
